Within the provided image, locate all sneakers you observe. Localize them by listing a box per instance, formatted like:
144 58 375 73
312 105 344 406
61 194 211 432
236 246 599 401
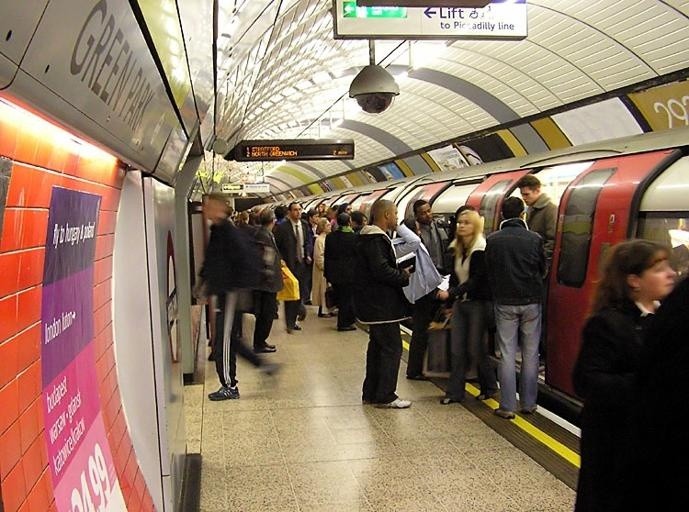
440 396 463 404
494 407 515 419
208 385 241 401
521 406 539 415
362 392 412 408
273 300 357 331
475 393 494 401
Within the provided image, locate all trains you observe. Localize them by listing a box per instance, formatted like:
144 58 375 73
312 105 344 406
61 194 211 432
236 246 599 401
253 124 689 431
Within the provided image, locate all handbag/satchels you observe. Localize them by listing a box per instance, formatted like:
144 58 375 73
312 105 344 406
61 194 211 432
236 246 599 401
276 267 301 301
427 309 451 373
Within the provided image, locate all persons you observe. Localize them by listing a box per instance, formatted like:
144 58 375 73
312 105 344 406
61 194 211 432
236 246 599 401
391 200 476 379
350 197 413 409
636 271 689 512
569 236 678 510
485 197 549 420
437 206 498 402
191 190 277 399
231 201 368 334
519 176 558 372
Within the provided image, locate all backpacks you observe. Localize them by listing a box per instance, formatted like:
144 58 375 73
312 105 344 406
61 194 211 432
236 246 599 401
243 241 278 292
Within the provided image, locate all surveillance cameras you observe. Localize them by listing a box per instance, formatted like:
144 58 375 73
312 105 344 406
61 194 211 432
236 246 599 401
348 65 402 115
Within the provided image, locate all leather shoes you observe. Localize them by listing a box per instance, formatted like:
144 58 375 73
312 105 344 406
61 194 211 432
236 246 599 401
262 342 276 352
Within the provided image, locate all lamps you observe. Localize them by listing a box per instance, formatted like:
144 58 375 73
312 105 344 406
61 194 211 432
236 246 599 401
348 39 400 115
